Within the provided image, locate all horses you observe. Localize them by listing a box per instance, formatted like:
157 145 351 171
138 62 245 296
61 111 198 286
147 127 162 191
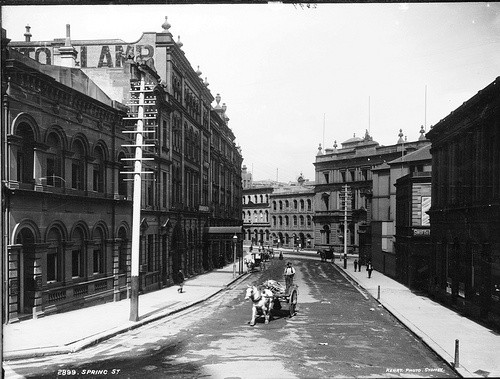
243 256 256 273
244 283 274 324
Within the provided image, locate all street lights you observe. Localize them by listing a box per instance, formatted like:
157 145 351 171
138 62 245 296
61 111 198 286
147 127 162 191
232 232 238 278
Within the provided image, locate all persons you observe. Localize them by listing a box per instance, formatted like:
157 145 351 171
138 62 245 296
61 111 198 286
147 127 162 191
358 258 362 272
176 269 185 293
283 262 296 287
354 258 358 272
367 261 373 279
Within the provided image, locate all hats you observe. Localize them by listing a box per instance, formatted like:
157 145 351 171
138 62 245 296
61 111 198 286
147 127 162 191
286 262 292 265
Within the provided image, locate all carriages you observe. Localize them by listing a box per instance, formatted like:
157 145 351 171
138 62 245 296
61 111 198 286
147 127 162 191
317 247 334 262
245 282 297 325
